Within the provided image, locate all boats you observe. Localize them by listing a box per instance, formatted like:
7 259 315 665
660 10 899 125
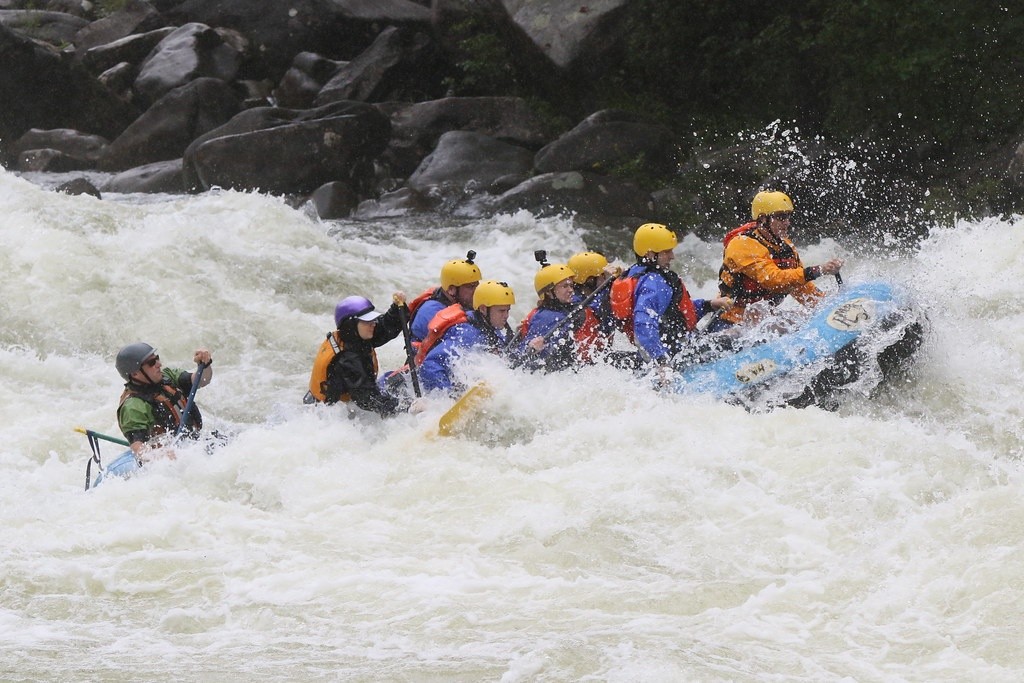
670 282 928 418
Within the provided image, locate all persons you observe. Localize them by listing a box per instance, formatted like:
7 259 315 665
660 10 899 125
406 260 482 352
307 291 426 414
515 250 624 373
711 189 845 330
610 223 743 372
115 342 212 461
414 281 516 395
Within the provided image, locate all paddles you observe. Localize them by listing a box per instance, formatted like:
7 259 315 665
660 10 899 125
158 358 205 470
391 293 424 402
646 291 736 401
436 267 625 432
71 426 132 448
428 323 525 441
833 264 849 290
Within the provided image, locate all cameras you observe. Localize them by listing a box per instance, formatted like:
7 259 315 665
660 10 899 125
535 250 547 261
466 250 476 259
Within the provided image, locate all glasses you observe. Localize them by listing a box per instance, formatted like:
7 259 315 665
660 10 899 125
141 354 161 368
766 213 794 222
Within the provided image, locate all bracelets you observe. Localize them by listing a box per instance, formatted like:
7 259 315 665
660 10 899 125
204 359 212 368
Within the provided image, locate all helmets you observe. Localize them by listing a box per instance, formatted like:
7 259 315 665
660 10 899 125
751 190 795 223
441 260 483 292
534 264 575 300
567 252 608 284
632 223 678 258
334 295 385 329
473 281 516 312
116 344 156 385
